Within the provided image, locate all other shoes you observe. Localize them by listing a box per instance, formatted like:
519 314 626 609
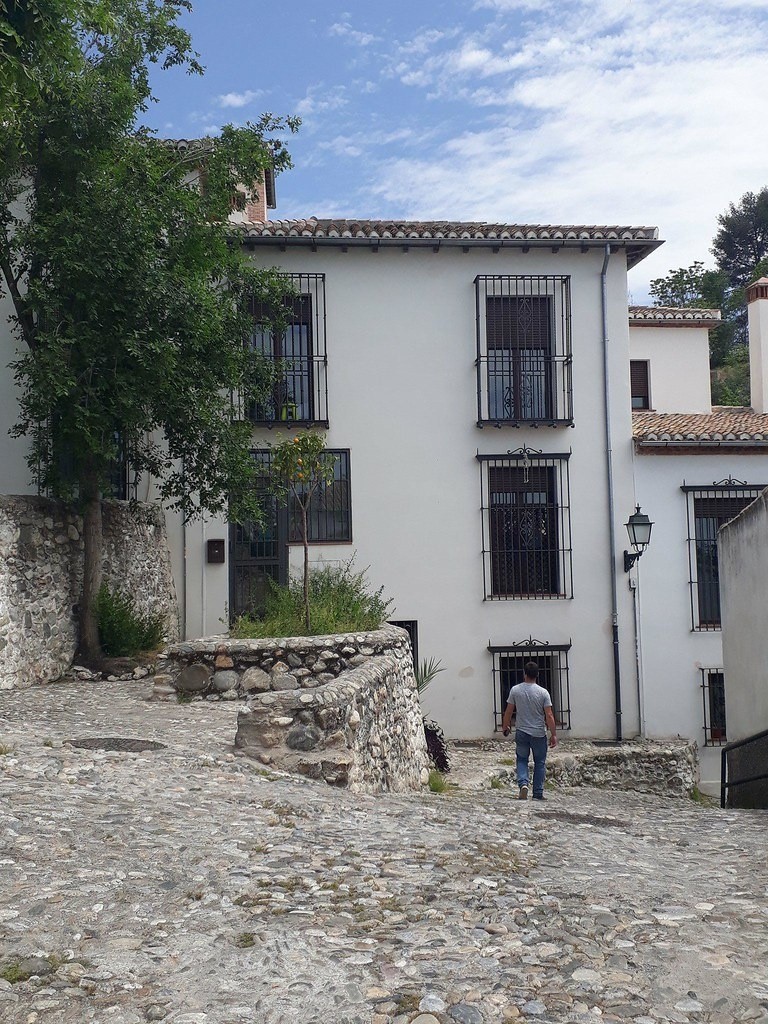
532 796 548 801
519 786 528 799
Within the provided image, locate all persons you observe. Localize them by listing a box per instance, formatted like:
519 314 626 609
503 662 557 800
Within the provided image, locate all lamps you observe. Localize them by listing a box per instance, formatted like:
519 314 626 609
622 503 655 573
